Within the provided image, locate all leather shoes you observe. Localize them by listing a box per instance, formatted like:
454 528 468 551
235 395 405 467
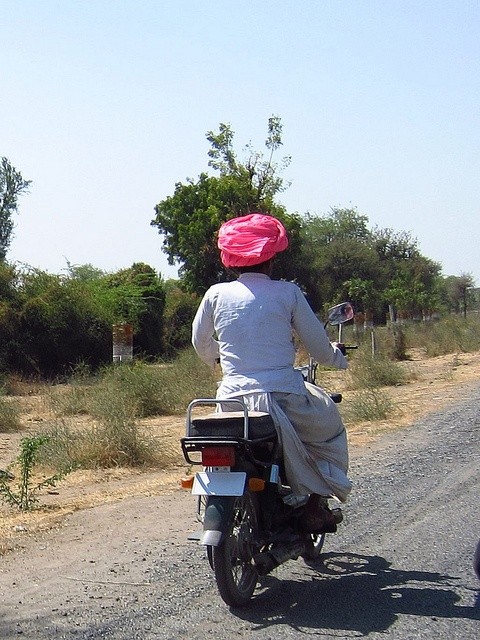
303 503 344 532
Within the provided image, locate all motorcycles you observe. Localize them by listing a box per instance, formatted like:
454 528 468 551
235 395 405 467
180 302 359 607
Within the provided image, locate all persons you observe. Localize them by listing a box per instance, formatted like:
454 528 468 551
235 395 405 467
191 214 352 532
